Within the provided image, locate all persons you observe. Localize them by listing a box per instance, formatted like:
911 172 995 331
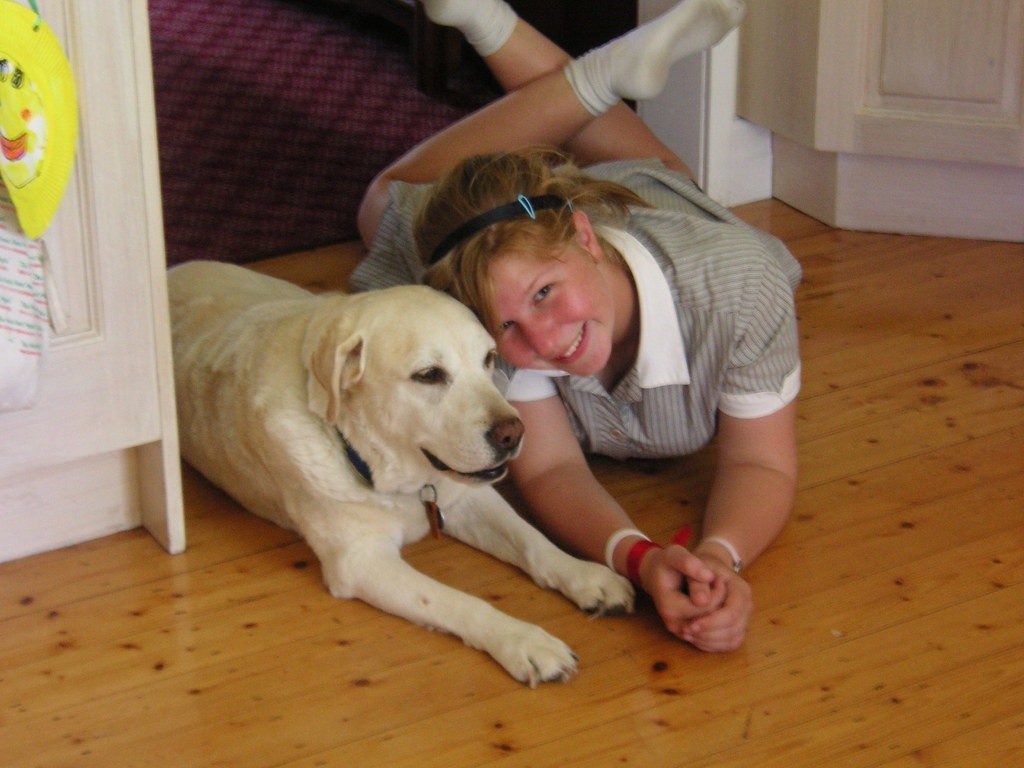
347 0 802 654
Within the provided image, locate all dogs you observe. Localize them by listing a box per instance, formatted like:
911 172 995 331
163 260 638 689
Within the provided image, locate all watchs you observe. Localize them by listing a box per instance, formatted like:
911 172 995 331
702 537 744 576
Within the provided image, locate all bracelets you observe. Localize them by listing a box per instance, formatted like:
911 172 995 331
626 525 693 591
602 528 652 573
